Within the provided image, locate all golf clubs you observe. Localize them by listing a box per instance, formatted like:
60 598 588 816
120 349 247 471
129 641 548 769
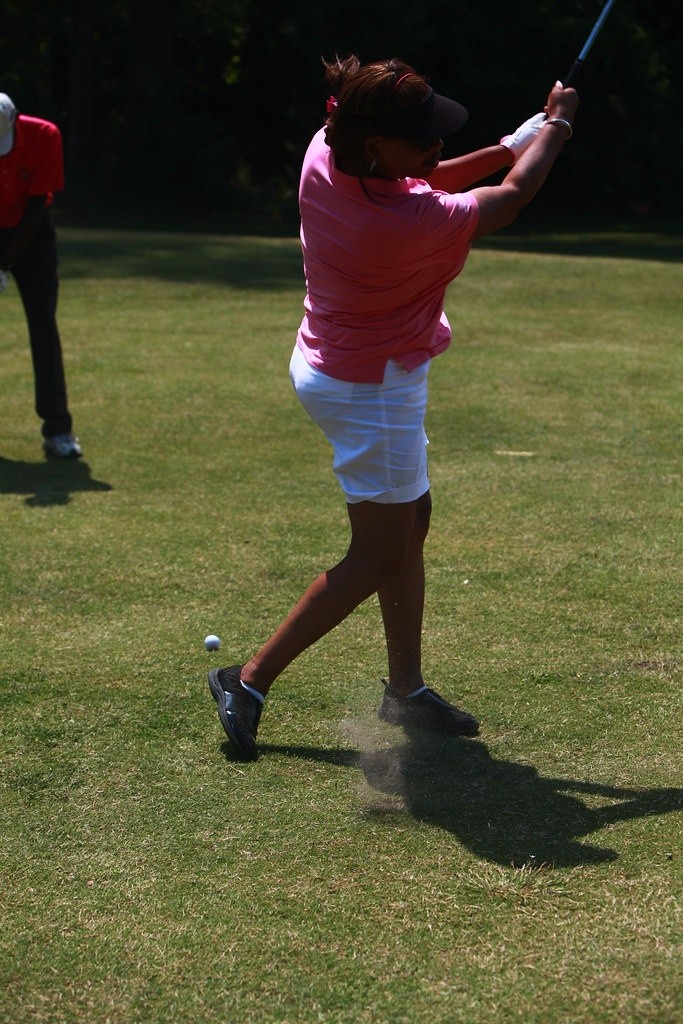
542 0 615 121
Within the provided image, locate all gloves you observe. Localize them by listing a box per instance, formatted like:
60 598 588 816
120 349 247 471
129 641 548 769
500 112 549 167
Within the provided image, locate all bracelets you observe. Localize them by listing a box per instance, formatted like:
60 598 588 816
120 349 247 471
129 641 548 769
545 119 573 140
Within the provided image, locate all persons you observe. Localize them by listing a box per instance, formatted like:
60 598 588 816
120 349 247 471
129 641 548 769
0 92 83 461
208 56 579 750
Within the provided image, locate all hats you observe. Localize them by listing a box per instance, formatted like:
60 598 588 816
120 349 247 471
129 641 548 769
343 66 469 140
0 92 19 156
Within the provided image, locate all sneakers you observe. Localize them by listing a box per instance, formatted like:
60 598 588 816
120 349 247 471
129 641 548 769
377 678 479 734
42 433 82 459
207 663 265 760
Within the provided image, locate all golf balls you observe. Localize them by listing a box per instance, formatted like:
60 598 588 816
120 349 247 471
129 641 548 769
204 635 220 650
